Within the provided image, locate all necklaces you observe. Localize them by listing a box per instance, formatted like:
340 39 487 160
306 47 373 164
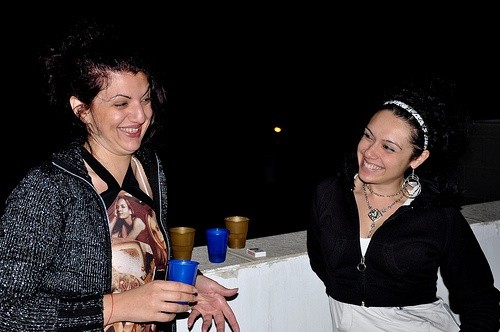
363 179 407 237
365 182 407 198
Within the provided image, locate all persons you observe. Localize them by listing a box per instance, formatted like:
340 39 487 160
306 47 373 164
145 208 168 264
0 51 240 332
308 89 500 332
109 194 157 265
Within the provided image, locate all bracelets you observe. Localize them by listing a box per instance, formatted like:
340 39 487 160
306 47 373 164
103 293 113 329
114 214 118 218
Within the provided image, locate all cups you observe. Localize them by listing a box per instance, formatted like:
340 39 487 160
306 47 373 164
168 260 200 304
169 227 195 260
206 228 228 263
225 216 249 249
